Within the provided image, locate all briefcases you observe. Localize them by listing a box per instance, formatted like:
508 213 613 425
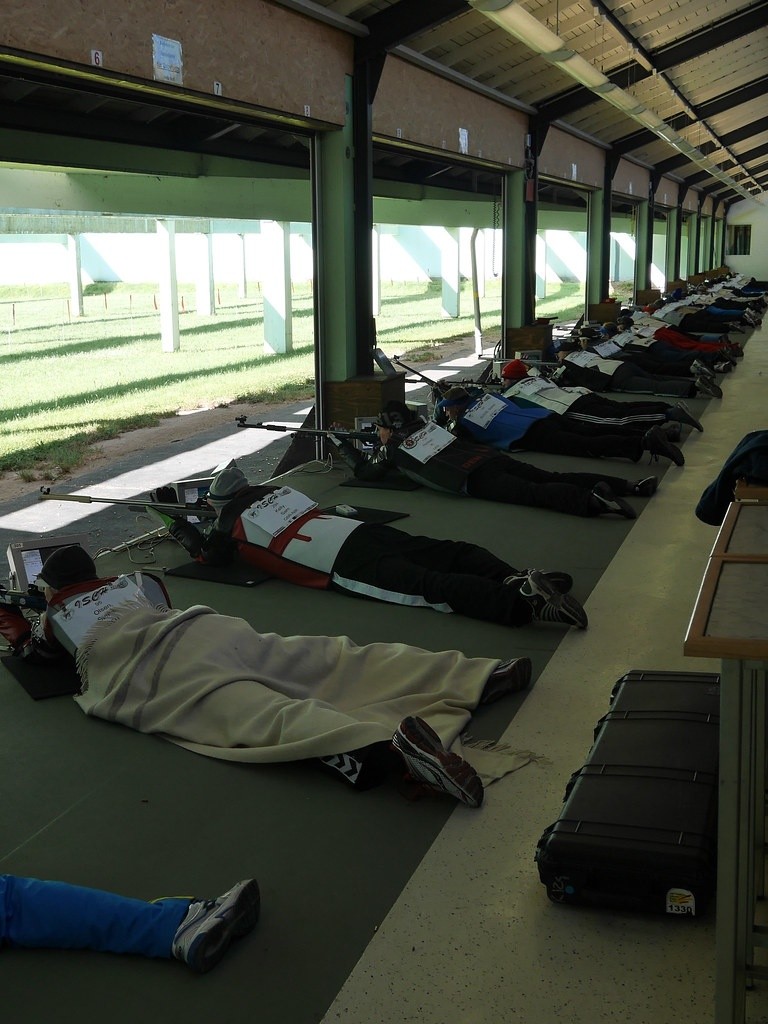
533 669 721 920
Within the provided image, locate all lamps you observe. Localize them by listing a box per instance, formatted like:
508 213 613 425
468 1 767 210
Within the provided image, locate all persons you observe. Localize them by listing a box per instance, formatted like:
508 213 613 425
148 467 588 630
329 402 657 520
0 546 532 806
433 274 768 466
0 876 260 969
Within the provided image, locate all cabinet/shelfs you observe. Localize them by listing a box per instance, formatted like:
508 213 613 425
704 270 719 279
506 318 550 362
636 289 661 306
688 275 706 287
588 301 622 322
717 267 729 274
322 371 408 459
667 280 687 292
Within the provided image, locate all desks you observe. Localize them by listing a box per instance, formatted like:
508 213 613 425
681 501 768 1023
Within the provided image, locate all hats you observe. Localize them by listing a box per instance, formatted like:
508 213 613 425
34 545 96 590
555 271 736 353
206 467 248 507
502 359 528 379
437 387 469 407
373 401 419 429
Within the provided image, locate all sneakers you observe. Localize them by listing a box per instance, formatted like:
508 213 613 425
642 423 682 441
587 474 659 521
689 292 768 400
667 402 703 432
171 876 261 975
481 656 532 705
642 425 685 466
390 716 484 809
502 567 589 630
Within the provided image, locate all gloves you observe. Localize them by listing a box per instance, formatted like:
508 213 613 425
433 406 449 427
326 421 348 447
145 486 187 531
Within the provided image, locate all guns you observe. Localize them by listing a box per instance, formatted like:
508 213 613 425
38 485 218 519
1 582 49 613
403 376 502 387
478 354 558 366
235 414 379 443
393 354 448 393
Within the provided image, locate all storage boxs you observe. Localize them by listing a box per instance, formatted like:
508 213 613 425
532 668 720 922
